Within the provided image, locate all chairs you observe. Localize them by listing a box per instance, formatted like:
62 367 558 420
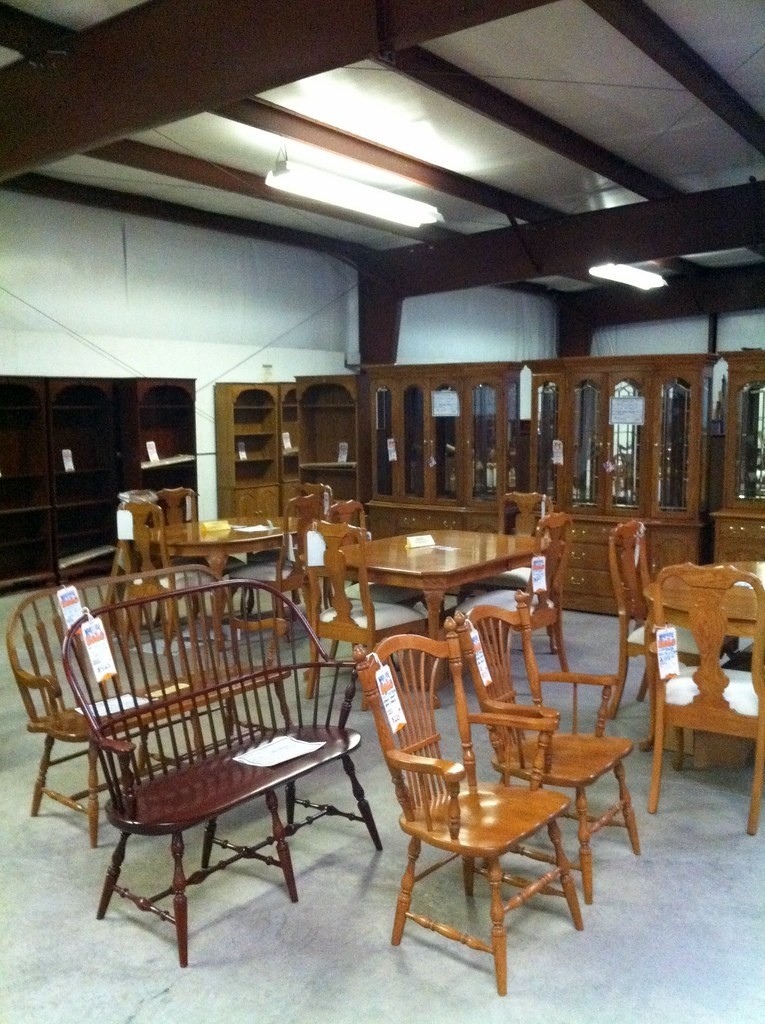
480 490 554 637
353 616 585 998
447 511 574 685
102 482 335 654
644 562 765 837
455 588 642 905
607 519 739 721
326 500 425 662
302 518 427 710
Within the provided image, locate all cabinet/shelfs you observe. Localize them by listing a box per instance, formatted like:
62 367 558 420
524 352 723 619
119 378 198 565
46 377 123 586
295 374 372 514
355 360 525 598
0 375 56 590
214 382 281 518
708 349 765 563
279 382 301 518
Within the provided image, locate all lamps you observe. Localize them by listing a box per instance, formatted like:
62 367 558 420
264 147 446 228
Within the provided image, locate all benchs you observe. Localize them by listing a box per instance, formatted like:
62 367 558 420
7 561 294 849
64 578 383 972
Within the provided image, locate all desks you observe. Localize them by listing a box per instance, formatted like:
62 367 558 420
134 518 318 652
339 529 550 706
